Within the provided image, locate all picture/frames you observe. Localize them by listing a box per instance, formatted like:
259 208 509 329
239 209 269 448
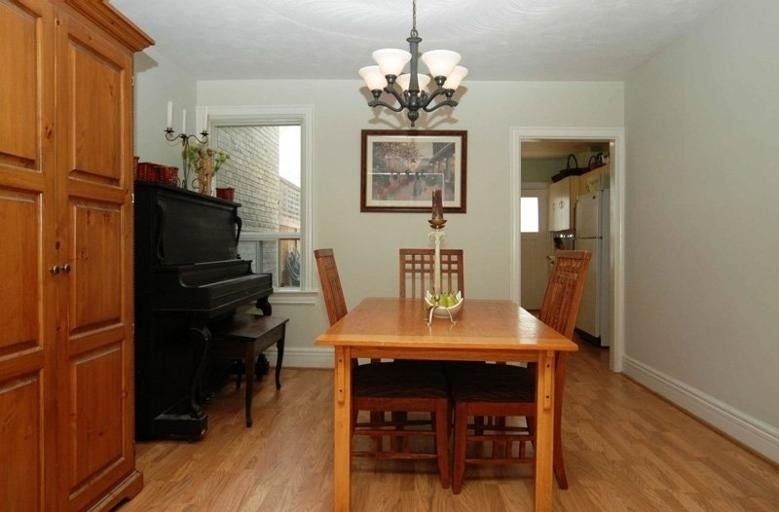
360 129 467 213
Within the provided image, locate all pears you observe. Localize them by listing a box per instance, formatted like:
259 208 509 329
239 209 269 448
430 287 459 307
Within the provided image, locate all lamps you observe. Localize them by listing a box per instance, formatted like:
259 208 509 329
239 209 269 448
424 190 456 326
357 0 468 127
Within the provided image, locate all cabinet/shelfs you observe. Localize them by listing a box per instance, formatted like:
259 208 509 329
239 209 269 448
0 0 156 512
548 175 578 232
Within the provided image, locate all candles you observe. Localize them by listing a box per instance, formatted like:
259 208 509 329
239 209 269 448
204 106 208 130
182 109 186 134
166 101 173 129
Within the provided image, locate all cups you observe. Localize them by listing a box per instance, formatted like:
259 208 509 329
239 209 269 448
216 188 234 203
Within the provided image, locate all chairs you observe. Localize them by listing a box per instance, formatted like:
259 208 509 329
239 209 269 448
313 250 450 489
452 249 592 495
399 249 464 299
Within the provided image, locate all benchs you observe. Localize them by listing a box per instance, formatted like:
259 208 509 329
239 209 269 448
212 313 289 427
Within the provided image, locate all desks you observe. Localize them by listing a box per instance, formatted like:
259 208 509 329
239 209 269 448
313 296 580 512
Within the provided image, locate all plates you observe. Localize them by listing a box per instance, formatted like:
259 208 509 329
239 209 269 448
424 291 464 319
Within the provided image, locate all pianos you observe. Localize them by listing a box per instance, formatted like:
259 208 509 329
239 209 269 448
133 178 272 443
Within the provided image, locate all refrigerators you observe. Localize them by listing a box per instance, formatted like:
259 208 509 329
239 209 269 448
574 168 603 338
